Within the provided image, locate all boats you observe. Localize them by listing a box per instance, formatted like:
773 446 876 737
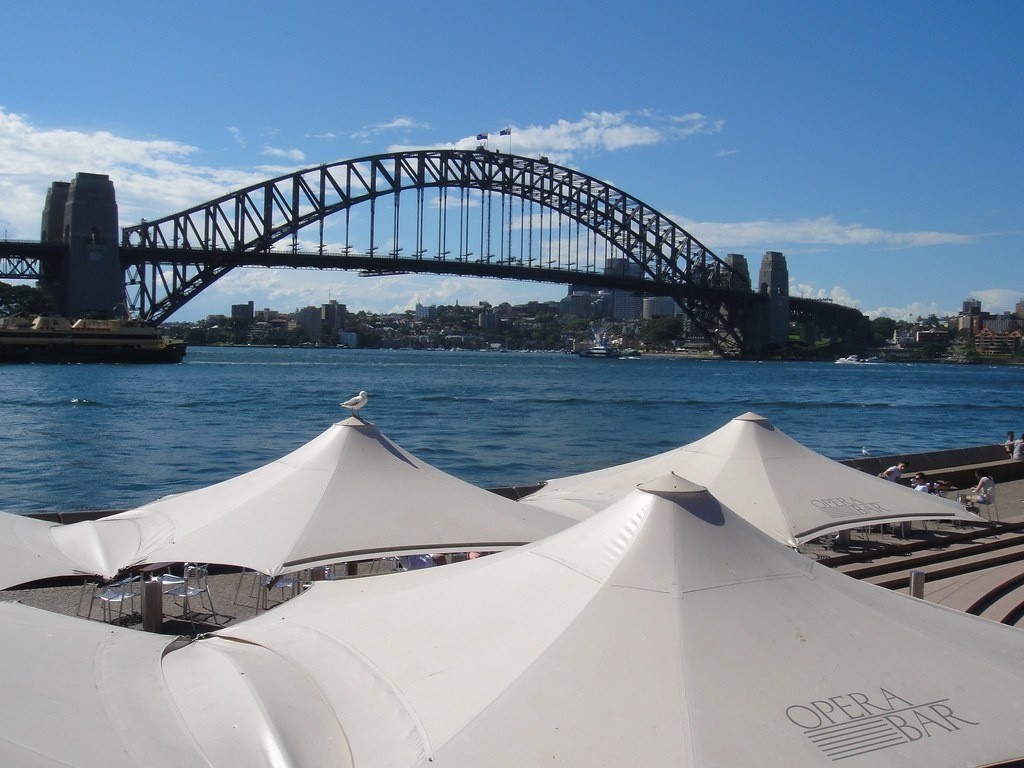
834 355 859 365
0 311 190 364
578 333 626 360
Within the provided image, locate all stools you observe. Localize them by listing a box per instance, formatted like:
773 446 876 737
847 518 963 543
232 556 401 617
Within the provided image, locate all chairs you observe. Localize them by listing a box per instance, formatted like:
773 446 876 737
76 563 218 632
973 488 1000 523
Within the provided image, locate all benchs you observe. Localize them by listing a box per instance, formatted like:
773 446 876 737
899 456 1024 491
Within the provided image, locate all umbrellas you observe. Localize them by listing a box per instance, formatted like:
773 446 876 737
0 407 1024 768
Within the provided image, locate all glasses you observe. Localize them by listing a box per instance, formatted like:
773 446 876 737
915 478 919 480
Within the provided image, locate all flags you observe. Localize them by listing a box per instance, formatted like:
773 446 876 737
477 132 488 140
500 128 511 136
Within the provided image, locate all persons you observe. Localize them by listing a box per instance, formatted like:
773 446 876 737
878 460 910 484
914 472 928 493
996 434 1024 460
966 468 995 505
1004 431 1015 458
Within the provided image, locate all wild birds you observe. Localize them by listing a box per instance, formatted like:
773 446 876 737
339 390 368 419
862 446 871 455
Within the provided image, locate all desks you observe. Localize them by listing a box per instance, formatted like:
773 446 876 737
77 565 139 620
929 484 959 501
136 562 178 604
